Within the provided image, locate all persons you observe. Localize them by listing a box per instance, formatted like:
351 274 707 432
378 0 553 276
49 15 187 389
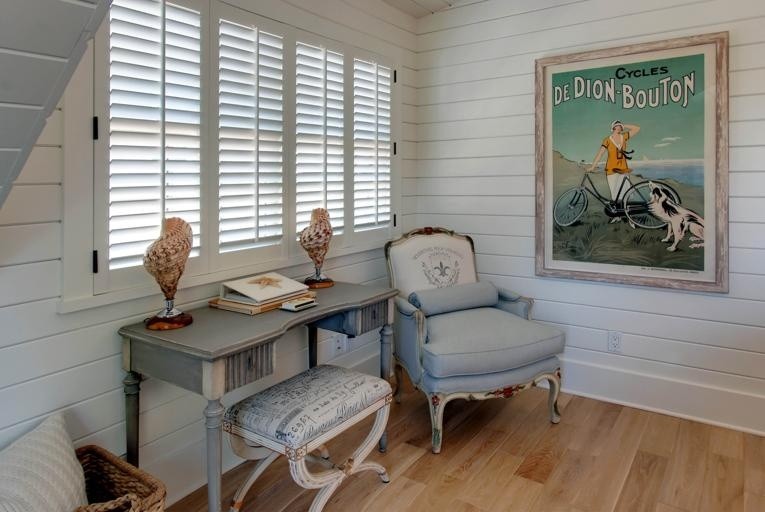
586 119 641 230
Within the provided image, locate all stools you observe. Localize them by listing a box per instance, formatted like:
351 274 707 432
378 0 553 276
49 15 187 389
223 363 394 512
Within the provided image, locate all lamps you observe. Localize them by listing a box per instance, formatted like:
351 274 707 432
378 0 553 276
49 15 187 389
145 217 194 331
300 208 335 288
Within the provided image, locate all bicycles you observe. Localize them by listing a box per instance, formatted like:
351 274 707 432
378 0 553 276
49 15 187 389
554 159 680 230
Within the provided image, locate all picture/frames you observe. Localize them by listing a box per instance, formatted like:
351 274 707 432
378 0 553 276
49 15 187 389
534 30 729 294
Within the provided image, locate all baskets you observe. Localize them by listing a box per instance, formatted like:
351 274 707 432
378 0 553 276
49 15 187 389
73 444 167 511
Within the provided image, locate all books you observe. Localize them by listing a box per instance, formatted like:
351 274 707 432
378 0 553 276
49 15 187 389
207 270 317 317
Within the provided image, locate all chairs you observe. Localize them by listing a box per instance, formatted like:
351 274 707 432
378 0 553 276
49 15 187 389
384 227 565 454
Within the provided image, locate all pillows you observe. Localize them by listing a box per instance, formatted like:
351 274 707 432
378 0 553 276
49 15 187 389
0 413 89 512
409 280 499 316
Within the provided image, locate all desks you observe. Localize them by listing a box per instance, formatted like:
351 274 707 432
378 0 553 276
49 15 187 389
117 281 400 512
